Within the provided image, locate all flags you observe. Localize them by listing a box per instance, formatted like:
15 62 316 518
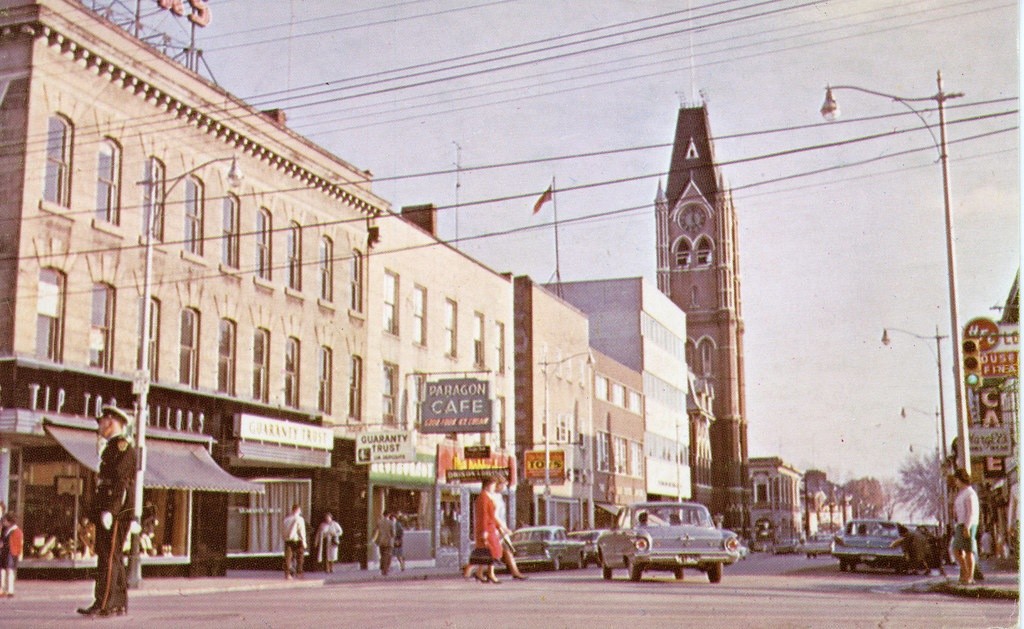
532 184 551 215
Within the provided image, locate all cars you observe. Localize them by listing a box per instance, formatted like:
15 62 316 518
772 539 804 556
738 537 750 561
597 502 741 584
565 529 612 566
830 519 918 574
806 535 834 558
904 523 946 567
499 524 588 572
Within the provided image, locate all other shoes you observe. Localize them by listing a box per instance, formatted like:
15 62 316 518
108 605 127 613
0 593 4 597
77 605 108 616
7 594 13 597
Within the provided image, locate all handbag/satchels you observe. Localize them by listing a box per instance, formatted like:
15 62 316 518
470 548 494 564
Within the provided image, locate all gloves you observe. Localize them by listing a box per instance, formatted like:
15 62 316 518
102 512 113 529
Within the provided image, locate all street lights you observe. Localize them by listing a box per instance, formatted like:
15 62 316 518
118 153 244 596
878 325 956 565
536 348 597 525
818 70 973 483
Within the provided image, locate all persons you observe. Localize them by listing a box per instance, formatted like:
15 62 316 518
669 513 681 524
917 526 946 577
390 514 405 571
38 535 56 560
981 527 994 555
638 512 647 526
899 524 931 575
1006 483 1020 557
142 498 156 534
371 509 396 575
0 501 23 596
462 476 513 584
76 406 138 617
80 516 96 557
473 472 529 582
282 504 307 580
952 469 979 585
314 512 343 573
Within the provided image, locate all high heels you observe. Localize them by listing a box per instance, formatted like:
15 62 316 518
473 573 488 582
459 566 467 580
487 574 500 583
513 574 528 579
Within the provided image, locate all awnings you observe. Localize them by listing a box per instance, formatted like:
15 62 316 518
44 424 266 493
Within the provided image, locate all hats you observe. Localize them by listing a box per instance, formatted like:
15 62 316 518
95 406 128 424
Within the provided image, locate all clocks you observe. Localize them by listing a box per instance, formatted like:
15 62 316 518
679 204 707 232
